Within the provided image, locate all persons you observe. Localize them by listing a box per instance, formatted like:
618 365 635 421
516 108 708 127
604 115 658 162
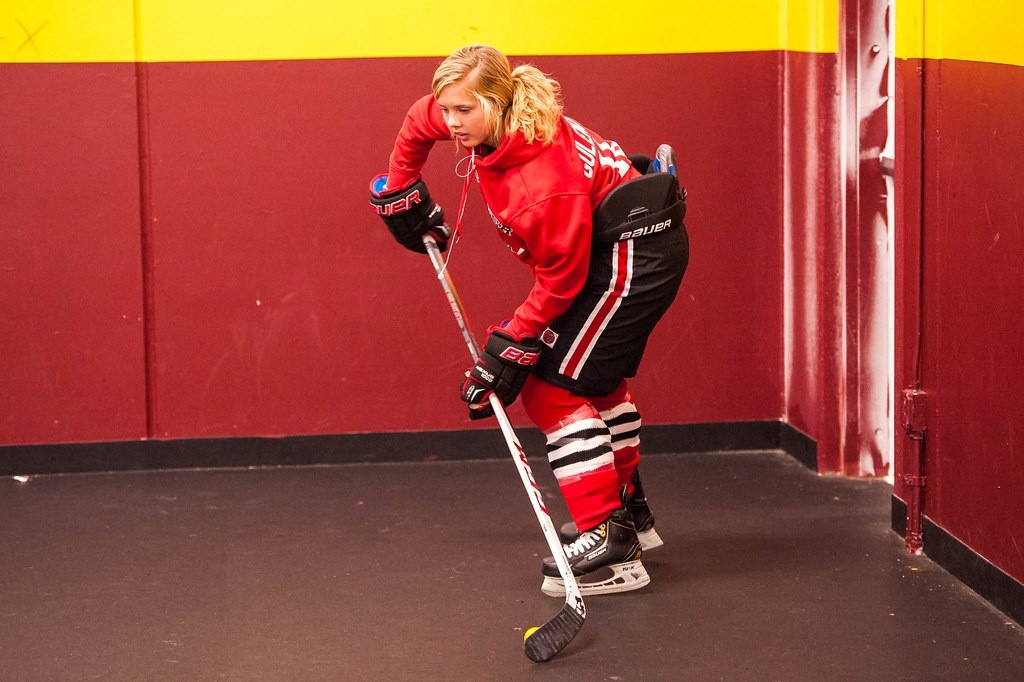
369 45 689 598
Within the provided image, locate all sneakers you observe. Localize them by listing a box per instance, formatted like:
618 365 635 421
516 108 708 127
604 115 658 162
560 468 663 552
540 484 650 597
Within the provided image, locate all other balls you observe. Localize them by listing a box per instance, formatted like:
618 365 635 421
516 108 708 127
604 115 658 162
524 626 540 641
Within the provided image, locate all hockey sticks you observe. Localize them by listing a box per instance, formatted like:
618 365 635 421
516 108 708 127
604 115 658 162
425 233 588 663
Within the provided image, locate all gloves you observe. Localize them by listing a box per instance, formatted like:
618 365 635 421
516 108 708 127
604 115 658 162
459 318 543 420
369 173 451 254
